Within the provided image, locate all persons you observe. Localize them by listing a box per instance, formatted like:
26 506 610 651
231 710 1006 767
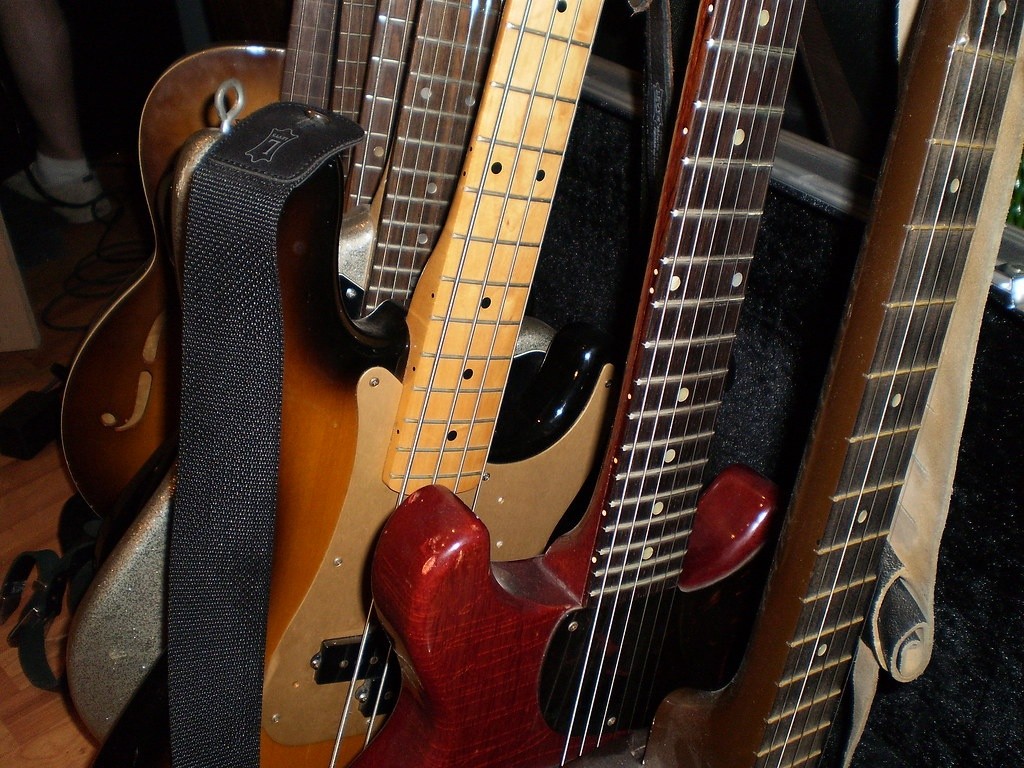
0 0 111 221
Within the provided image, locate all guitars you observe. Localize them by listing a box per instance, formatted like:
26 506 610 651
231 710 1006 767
57 0 1023 768
